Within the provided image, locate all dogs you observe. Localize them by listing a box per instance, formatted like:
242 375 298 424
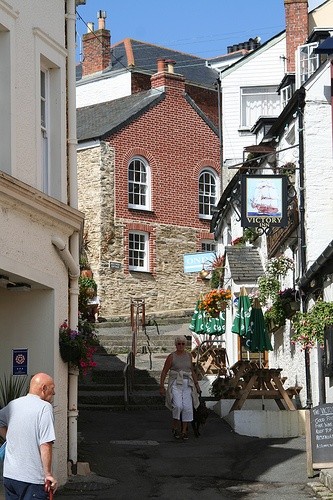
193 396 208 437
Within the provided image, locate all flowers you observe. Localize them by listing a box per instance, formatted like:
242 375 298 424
59 322 100 379
198 227 333 354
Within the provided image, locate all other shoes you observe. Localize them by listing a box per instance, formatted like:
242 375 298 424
175 433 180 438
182 433 188 439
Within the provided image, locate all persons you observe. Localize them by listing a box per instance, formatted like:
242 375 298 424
0 373 58 500
160 336 202 440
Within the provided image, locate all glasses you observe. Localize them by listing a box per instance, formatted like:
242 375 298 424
178 343 185 345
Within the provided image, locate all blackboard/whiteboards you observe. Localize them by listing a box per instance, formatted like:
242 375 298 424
309 402 333 469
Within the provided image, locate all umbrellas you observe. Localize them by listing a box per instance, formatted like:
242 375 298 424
189 287 270 410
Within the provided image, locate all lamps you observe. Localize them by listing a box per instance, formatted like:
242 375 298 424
199 260 215 279
